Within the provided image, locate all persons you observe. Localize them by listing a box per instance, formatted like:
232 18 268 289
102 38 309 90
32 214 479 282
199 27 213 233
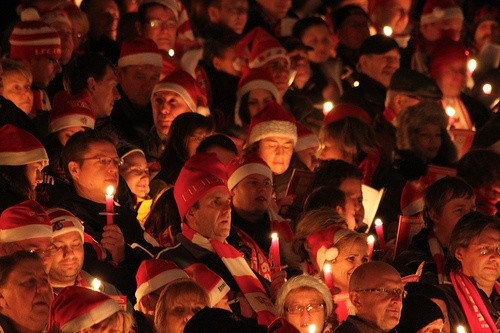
0 0 500 333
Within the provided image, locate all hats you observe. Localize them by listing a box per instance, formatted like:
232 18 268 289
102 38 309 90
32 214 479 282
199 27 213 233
421 0 500 76
150 69 211 116
400 181 426 217
0 200 84 244
174 151 272 224
48 285 121 333
275 275 334 321
9 0 90 58
114 135 146 160
247 102 321 151
117 36 163 68
324 103 371 128
134 259 230 312
396 290 445 333
235 26 314 126
333 0 443 99
0 90 95 167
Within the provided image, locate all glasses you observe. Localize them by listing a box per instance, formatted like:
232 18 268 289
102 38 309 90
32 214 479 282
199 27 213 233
48 56 64 65
469 240 500 255
71 31 82 40
355 286 408 299
15 242 63 259
80 155 122 167
284 301 324 314
142 18 177 30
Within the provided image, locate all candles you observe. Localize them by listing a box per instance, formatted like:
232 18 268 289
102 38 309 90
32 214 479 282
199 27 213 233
373 217 386 250
105 185 114 226
270 231 281 272
367 234 375 261
465 58 477 78
322 102 334 116
446 105 455 130
323 262 334 294
